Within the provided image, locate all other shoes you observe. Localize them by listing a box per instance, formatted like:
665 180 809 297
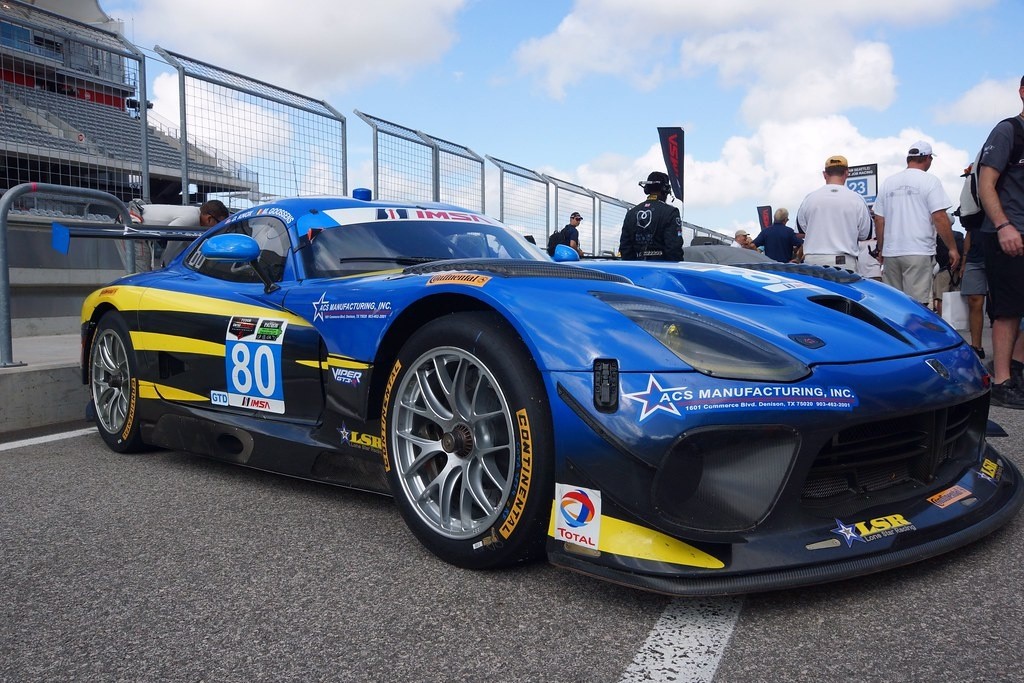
971 346 987 359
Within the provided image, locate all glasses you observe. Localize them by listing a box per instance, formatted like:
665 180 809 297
787 219 789 221
208 211 221 223
576 219 581 221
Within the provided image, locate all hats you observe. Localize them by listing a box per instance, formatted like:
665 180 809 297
824 155 848 166
908 141 937 159
570 212 583 220
735 230 750 236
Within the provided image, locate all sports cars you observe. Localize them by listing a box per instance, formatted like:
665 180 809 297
80 188 1022 596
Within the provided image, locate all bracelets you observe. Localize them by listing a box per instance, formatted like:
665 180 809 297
995 221 1011 233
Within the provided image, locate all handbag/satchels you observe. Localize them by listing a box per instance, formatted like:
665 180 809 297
942 274 984 332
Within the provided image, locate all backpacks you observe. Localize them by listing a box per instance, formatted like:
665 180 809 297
952 117 1023 229
547 230 566 257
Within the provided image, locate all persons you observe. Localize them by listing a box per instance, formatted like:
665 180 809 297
112 199 229 276
561 212 583 259
977 75 1024 410
934 212 964 316
857 217 881 278
732 208 806 263
871 140 960 310
794 156 873 273
619 171 684 262
959 230 990 359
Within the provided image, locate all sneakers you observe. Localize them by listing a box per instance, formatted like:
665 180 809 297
991 367 1024 409
987 353 1024 391
